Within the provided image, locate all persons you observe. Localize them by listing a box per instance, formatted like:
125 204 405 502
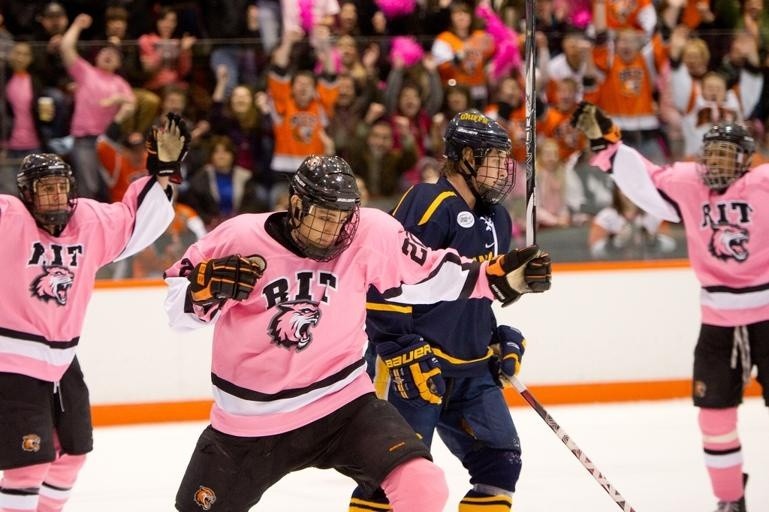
162 154 550 512
0 112 192 512
567 100 769 511
349 108 526 512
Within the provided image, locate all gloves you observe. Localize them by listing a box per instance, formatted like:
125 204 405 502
371 337 447 408
183 254 263 305
486 325 527 390
483 243 554 309
145 111 191 185
570 98 621 153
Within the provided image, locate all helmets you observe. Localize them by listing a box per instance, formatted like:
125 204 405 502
16 152 80 227
288 154 362 261
442 112 515 205
694 123 758 190
